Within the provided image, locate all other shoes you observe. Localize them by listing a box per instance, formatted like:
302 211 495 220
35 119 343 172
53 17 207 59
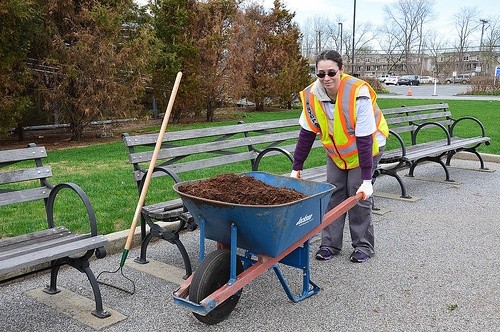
349 249 369 262
316 249 333 260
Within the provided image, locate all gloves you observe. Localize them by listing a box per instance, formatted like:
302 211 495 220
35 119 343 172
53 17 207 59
289 170 299 179
357 179 373 200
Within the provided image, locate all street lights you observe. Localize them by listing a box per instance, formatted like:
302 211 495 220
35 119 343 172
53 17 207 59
338 22 343 53
477 20 489 61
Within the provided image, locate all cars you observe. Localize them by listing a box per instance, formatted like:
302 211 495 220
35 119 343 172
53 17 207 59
419 75 440 84
375 73 401 86
397 75 419 87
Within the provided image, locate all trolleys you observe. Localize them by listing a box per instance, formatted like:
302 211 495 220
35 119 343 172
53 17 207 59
166 171 364 324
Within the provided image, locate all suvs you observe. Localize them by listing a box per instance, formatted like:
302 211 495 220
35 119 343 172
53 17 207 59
446 73 473 83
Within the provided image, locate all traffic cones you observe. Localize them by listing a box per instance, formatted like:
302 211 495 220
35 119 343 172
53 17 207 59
407 85 413 96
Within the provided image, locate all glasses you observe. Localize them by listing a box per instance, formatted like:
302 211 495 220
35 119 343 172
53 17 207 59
316 68 340 78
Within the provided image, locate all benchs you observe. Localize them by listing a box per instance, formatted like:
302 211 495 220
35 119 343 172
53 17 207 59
0 142 128 331
120 119 380 286
371 102 490 202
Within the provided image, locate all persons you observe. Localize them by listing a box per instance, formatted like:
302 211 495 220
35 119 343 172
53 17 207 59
290 50 389 263
